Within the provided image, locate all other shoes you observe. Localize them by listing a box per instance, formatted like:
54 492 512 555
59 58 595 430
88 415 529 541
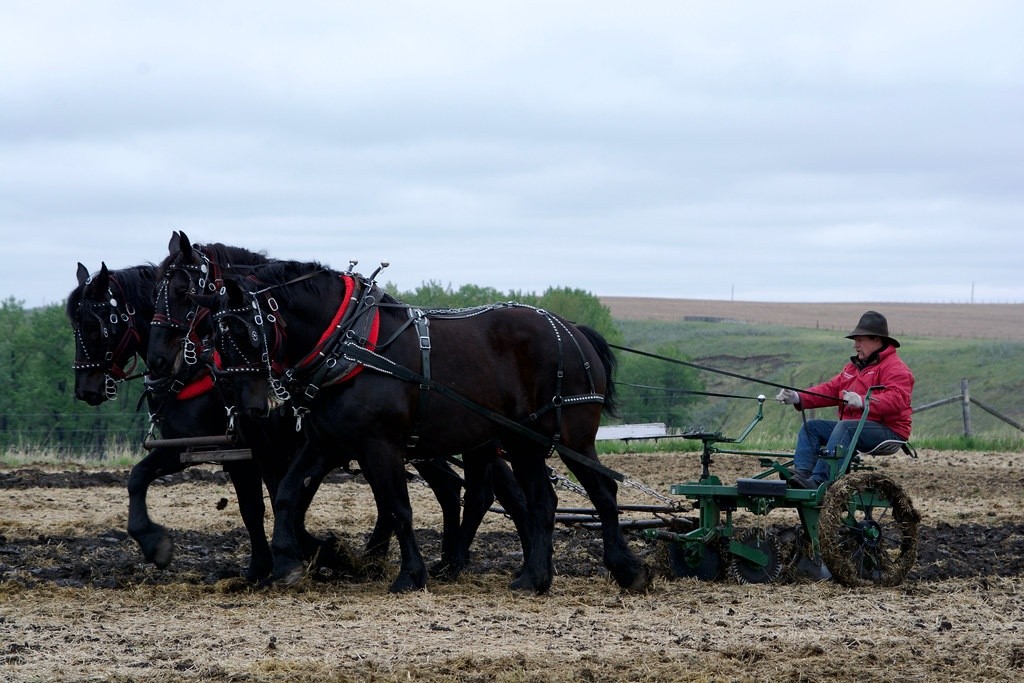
789 472 819 490
780 466 807 479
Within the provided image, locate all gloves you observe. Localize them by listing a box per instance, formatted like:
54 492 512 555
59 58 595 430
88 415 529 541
842 390 863 408
777 388 799 405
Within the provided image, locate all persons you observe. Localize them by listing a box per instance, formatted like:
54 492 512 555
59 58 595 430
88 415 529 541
777 310 915 491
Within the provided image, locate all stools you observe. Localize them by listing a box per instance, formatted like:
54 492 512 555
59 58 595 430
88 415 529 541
856 439 907 457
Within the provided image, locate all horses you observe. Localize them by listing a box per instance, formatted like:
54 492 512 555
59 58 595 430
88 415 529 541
68 227 656 598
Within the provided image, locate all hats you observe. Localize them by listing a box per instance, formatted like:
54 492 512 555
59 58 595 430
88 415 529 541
844 311 901 348
